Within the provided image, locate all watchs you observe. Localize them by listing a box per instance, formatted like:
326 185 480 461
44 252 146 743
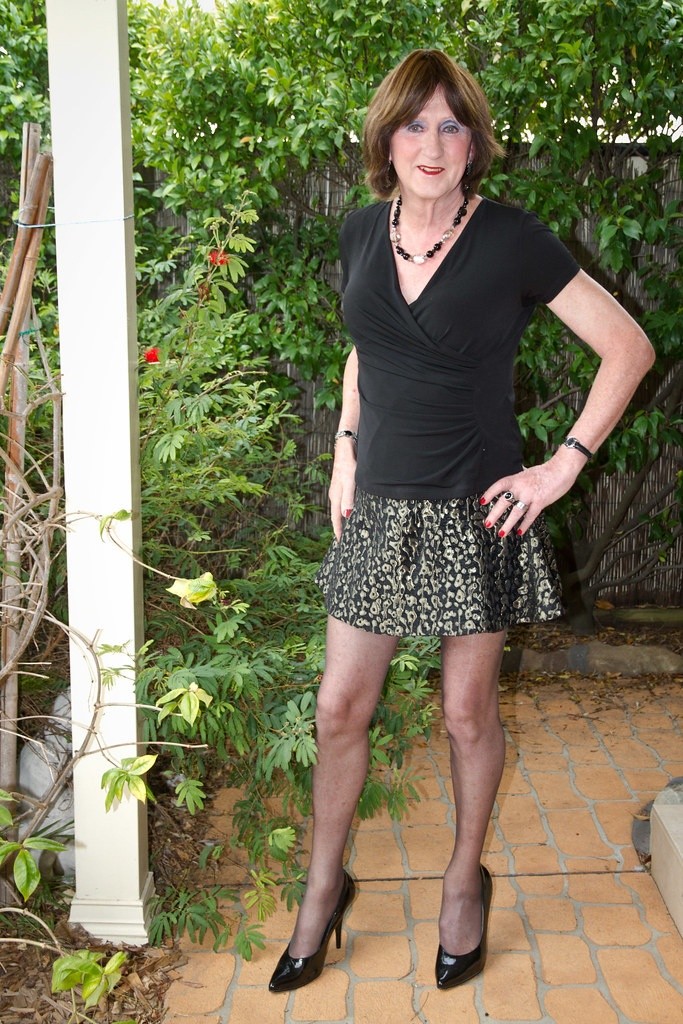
562 437 593 461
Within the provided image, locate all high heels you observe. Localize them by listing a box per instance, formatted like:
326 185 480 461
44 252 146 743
433 863 493 989
268 867 358 993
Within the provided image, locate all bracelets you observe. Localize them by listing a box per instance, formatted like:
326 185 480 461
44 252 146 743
334 429 358 445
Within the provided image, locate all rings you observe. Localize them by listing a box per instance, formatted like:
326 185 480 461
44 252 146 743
513 500 528 512
503 491 516 504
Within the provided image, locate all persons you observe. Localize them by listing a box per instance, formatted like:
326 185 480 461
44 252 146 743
263 48 659 994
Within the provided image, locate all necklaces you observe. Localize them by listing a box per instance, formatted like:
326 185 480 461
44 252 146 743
388 182 470 266
